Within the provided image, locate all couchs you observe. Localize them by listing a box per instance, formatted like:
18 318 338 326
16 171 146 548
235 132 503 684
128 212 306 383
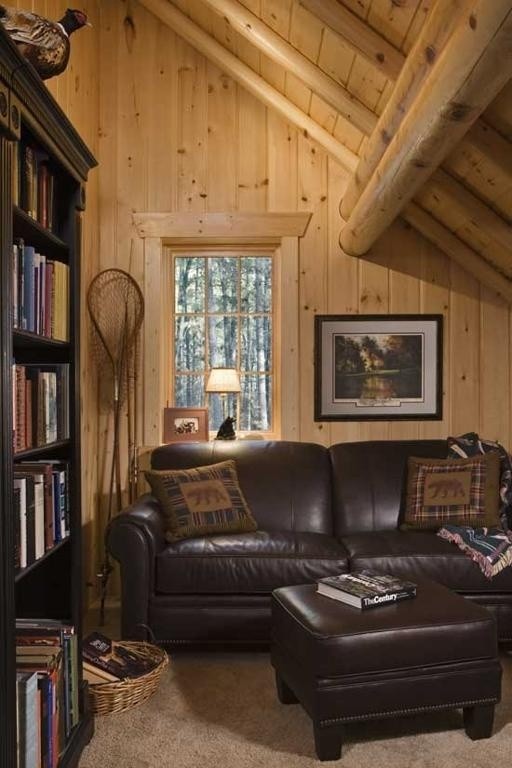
103 440 511 652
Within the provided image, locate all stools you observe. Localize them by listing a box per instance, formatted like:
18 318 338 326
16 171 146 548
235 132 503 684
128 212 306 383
270 573 502 760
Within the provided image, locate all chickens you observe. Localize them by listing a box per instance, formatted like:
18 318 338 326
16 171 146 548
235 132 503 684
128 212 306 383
0 7 96 82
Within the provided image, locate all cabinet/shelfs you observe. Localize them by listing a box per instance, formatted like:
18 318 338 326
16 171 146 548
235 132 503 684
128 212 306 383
0 21 97 768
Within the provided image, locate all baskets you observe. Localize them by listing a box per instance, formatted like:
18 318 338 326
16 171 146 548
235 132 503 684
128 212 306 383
80 636 170 715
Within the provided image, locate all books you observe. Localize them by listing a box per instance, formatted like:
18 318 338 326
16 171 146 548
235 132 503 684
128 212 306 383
80 631 158 682
13 459 70 569
16 618 79 768
11 139 53 231
12 361 69 452
314 568 417 611
13 237 69 342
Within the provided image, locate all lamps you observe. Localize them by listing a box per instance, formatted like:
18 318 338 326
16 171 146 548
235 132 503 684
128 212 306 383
204 367 242 441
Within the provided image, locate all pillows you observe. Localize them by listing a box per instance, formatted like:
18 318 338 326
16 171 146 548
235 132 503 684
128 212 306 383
144 459 259 543
399 450 504 533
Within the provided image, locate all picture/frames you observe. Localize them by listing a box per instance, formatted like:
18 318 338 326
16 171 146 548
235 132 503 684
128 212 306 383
163 408 209 445
313 315 444 421
